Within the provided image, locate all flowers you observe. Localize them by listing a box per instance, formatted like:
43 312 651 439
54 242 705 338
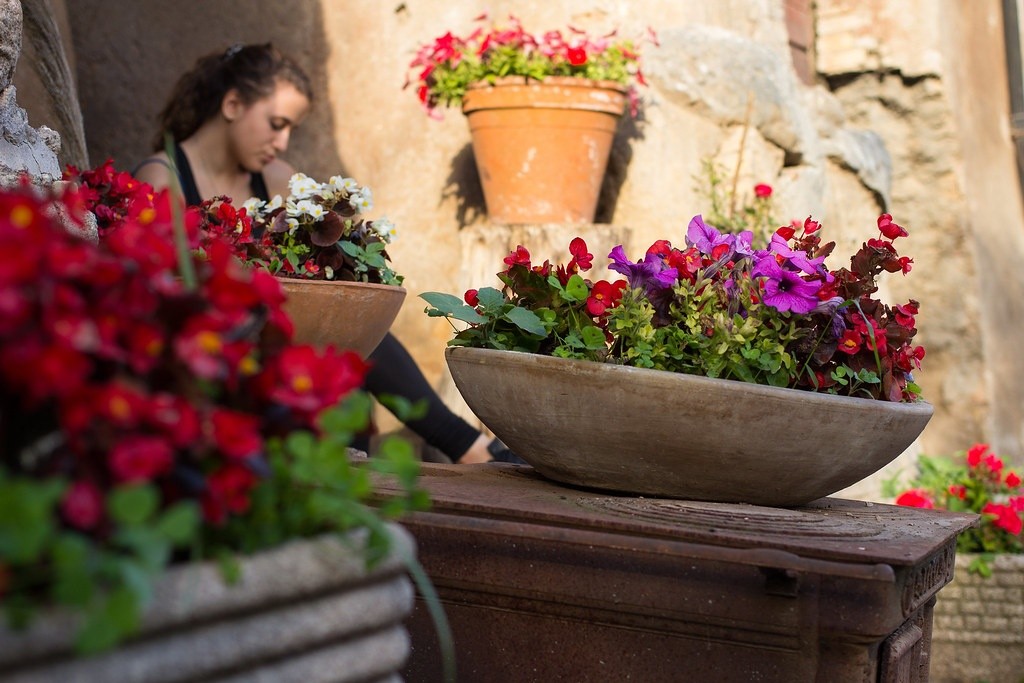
419 211 926 407
200 171 402 289
879 441 1024 579
403 13 661 119
0 157 432 655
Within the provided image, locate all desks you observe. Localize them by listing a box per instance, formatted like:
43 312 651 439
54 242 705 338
353 461 981 682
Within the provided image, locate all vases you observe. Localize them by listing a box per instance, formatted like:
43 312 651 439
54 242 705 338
274 276 407 365
0 520 421 683
442 346 935 509
460 75 630 226
931 554 1024 682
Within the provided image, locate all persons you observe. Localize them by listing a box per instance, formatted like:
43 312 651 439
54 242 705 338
132 42 527 464
